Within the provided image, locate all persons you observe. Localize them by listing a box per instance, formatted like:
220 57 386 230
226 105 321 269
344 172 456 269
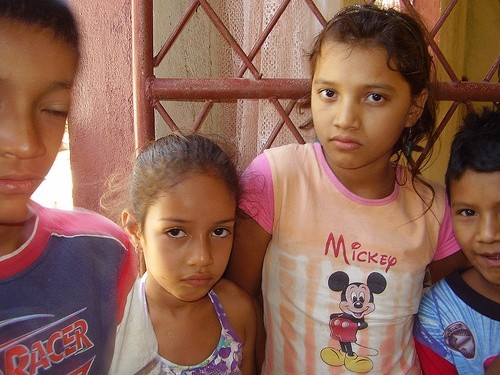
409 107 500 375
227 2 471 375
0 0 147 375
96 132 257 375
